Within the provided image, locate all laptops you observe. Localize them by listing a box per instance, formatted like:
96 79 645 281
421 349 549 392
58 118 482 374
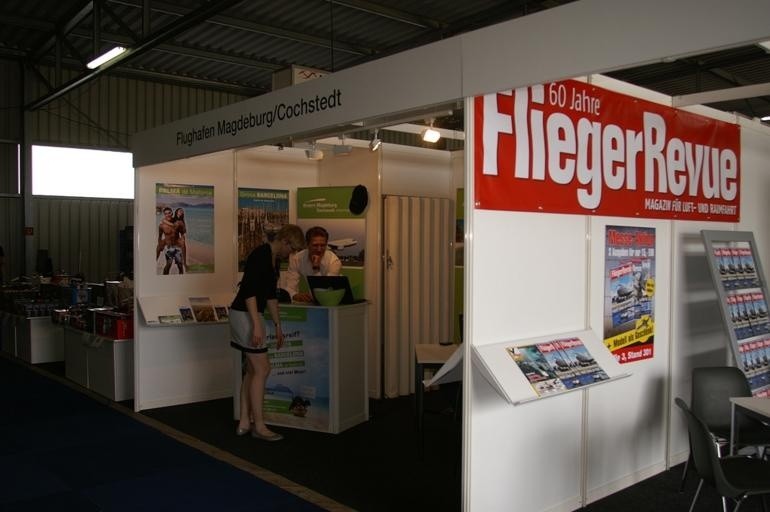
307 275 366 306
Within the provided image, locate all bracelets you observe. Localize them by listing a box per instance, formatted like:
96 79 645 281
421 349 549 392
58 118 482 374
275 322 280 327
312 264 321 273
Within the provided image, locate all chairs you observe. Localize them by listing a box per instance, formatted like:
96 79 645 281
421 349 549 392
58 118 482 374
673 394 769 511
681 366 756 492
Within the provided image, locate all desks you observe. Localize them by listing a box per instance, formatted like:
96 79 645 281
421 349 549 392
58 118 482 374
728 394 769 464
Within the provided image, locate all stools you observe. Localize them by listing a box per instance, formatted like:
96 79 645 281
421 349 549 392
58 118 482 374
412 340 463 453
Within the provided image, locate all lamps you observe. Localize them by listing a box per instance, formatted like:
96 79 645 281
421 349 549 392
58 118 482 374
303 118 442 162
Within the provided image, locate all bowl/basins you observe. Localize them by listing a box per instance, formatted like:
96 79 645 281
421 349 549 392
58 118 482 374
314 287 345 307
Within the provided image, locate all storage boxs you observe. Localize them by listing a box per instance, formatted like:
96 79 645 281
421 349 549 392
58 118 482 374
95 310 134 339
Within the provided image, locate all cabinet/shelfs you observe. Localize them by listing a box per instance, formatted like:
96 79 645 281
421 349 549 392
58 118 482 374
0 313 66 365
64 327 134 401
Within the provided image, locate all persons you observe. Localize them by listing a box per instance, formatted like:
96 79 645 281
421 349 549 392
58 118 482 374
158 207 184 275
285 226 341 303
156 208 189 270
227 224 305 440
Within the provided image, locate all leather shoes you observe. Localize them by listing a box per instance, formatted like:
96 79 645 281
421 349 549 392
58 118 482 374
236 426 285 441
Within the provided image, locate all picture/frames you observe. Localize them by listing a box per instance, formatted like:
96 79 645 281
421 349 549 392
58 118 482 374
159 294 234 324
